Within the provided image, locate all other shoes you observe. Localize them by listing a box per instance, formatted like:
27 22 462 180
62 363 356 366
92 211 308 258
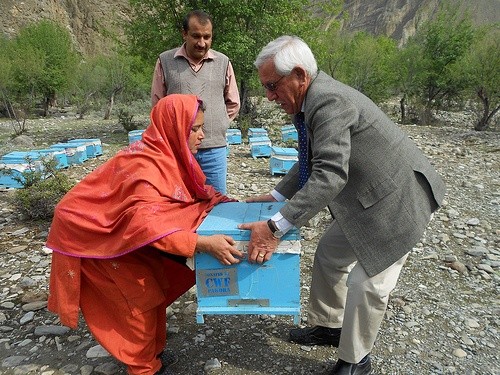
158 351 178 374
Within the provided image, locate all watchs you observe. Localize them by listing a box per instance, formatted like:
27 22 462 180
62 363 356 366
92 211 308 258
267 219 283 239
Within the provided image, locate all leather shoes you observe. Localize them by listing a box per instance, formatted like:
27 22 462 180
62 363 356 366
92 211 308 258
328 356 373 375
290 325 342 346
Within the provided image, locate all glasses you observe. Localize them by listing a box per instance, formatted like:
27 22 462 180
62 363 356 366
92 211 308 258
262 75 285 92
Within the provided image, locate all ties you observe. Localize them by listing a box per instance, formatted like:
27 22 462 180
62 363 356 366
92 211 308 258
296 112 308 189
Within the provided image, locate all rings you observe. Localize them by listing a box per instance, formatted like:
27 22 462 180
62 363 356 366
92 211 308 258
259 254 264 257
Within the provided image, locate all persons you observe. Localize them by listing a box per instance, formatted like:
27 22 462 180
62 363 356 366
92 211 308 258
151 9 241 195
238 35 445 375
45 94 243 375
